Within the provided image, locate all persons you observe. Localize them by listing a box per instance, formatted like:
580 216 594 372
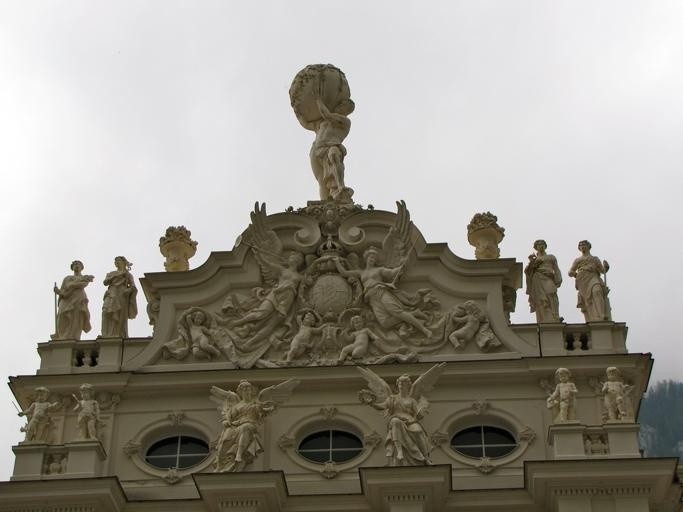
97 256 136 338
524 239 563 322
337 315 377 363
600 366 629 420
73 382 100 440
568 240 609 322
215 380 262 473
18 386 61 442
295 80 356 204
280 312 328 364
224 245 305 352
448 300 483 350
332 249 434 340
547 367 578 421
51 259 89 340
183 310 221 361
369 374 432 466
